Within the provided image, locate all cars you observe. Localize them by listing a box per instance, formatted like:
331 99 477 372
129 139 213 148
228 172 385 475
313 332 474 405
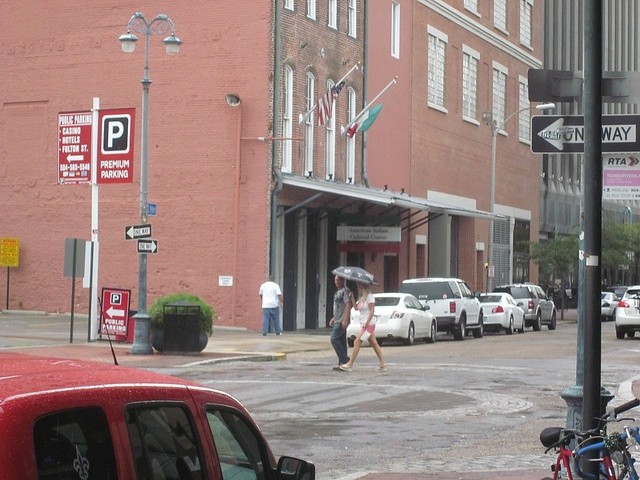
601 292 617 318
478 292 526 334
346 292 437 347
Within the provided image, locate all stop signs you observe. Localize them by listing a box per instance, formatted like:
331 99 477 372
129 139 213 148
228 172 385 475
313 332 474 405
97 108 135 182
101 288 130 341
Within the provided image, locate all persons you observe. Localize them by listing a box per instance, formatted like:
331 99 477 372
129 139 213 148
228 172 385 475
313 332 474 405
259 274 285 335
340 282 390 371
329 275 353 371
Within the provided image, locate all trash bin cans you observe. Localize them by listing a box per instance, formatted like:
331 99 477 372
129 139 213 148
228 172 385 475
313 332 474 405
162 302 200 356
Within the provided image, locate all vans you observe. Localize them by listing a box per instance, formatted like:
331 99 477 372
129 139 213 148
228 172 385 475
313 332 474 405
0 352 314 480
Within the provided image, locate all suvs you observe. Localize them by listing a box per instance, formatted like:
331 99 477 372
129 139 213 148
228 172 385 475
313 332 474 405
400 278 483 340
616 286 640 338
492 284 556 330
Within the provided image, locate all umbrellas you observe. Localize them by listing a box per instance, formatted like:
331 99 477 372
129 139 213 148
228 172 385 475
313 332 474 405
332 266 380 297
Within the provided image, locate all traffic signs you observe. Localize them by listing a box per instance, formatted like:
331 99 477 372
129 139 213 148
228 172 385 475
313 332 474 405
125 224 151 239
137 240 157 253
531 116 638 153
603 155 639 201
58 113 91 183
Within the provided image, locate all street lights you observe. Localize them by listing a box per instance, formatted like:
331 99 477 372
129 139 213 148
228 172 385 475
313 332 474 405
120 12 180 354
485 103 556 291
225 93 243 325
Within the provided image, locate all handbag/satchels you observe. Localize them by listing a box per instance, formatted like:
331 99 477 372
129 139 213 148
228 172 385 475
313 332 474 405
360 330 371 343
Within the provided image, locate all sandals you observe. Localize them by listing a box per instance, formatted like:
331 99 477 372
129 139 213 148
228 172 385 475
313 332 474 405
374 364 388 372
340 365 353 371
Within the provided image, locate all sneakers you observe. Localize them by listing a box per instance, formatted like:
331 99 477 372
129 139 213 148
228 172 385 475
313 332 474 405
333 359 350 370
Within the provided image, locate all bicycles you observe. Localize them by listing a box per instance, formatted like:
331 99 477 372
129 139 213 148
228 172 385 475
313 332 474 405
539 398 639 480
